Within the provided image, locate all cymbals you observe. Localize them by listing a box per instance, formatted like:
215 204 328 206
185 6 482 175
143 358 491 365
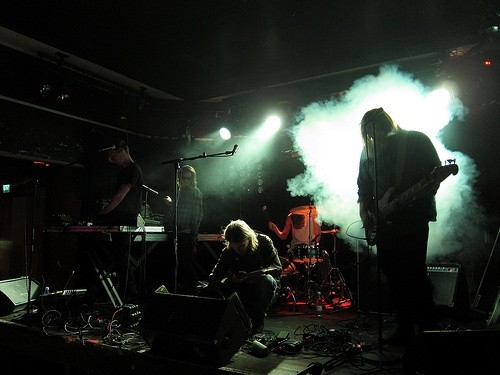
320 229 343 234
290 205 317 215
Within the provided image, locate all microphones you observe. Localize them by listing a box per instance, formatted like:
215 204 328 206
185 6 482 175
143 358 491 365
367 108 383 124
232 145 239 155
158 193 172 203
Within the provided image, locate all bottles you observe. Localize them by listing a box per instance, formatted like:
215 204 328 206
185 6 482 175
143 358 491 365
43 287 50 294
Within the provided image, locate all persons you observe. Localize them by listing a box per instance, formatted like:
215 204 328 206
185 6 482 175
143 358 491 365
357 106 444 323
269 196 321 288
162 164 203 293
200 220 282 335
95 138 144 304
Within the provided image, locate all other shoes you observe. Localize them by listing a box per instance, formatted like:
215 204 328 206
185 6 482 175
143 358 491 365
382 314 402 341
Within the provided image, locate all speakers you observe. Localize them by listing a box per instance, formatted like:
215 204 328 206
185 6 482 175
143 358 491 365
400 329 500 375
137 284 253 366
0 276 42 317
424 263 471 316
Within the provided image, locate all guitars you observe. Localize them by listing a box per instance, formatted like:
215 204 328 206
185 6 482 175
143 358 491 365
364 164 459 246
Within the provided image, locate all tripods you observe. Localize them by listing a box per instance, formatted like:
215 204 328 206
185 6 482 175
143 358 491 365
280 120 393 366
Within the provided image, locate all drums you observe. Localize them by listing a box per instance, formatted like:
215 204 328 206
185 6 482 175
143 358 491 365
290 241 322 262
279 256 292 275
311 266 353 313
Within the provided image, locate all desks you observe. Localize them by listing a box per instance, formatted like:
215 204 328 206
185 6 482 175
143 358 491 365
44 225 223 306
